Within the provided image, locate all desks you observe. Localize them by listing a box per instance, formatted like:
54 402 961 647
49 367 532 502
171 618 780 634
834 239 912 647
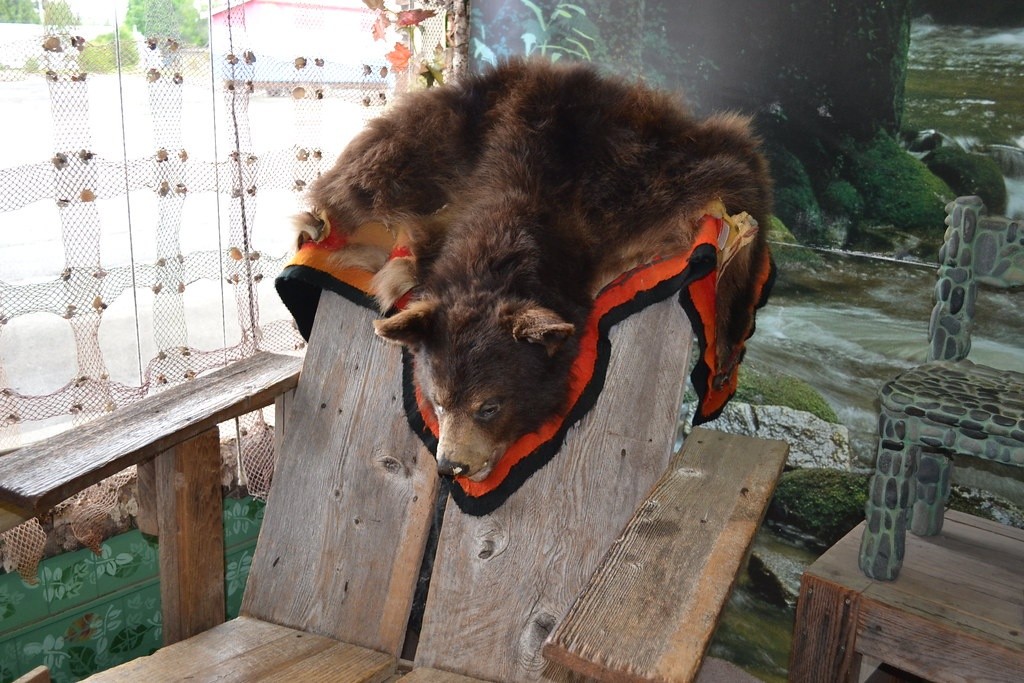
790 505 1024 683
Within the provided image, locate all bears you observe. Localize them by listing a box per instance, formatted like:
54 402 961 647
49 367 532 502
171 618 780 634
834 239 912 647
290 59 771 485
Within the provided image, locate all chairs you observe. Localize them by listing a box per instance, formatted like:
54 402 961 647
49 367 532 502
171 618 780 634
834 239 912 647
0 59 793 683
858 194 1024 581
